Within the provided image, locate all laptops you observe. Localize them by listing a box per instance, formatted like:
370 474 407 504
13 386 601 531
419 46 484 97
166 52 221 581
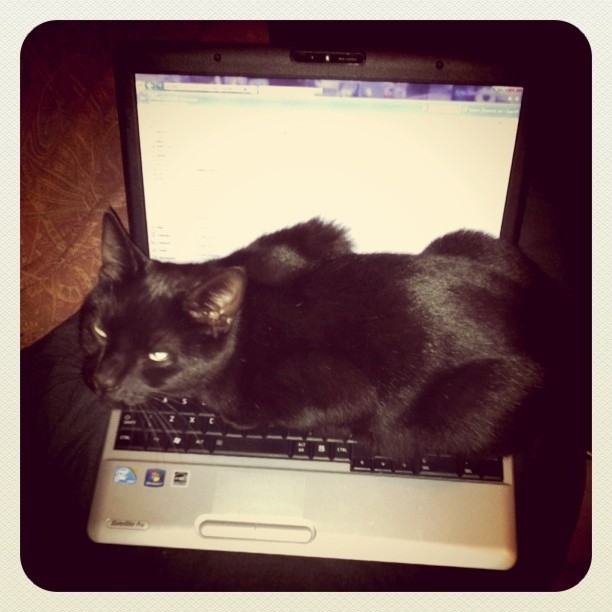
86 42 545 574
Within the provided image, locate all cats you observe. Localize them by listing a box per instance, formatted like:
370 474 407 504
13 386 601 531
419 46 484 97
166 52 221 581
78 204 551 469
